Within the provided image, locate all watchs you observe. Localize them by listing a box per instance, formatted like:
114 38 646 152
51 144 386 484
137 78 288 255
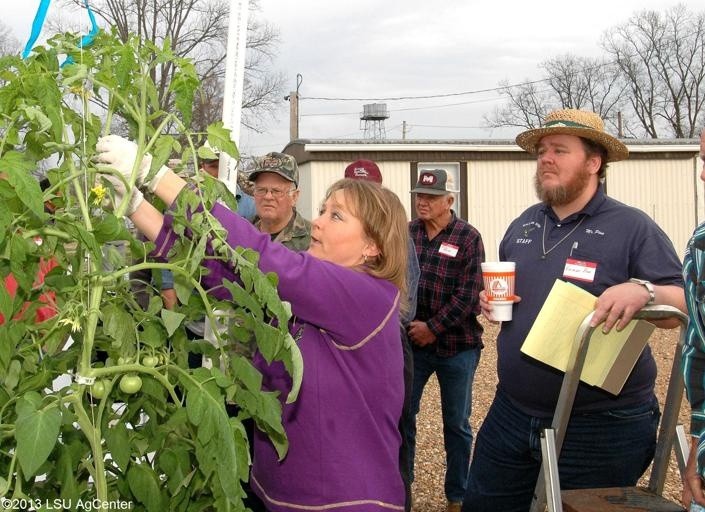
628 278 655 306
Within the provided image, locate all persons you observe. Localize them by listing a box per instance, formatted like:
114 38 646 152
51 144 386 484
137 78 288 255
459 111 694 512
681 120 705 512
0 113 409 508
399 166 484 511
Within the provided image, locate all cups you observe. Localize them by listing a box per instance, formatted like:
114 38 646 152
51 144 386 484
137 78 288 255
480 260 516 323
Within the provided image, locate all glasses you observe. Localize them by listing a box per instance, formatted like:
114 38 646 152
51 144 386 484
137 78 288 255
251 187 289 197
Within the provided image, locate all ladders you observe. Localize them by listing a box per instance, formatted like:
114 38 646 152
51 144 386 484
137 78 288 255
533 305 692 512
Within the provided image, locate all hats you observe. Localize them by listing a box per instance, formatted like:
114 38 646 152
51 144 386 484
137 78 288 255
345 159 382 184
409 170 455 195
249 152 299 189
516 109 629 162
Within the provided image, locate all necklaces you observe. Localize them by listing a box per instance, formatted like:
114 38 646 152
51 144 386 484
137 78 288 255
540 213 588 259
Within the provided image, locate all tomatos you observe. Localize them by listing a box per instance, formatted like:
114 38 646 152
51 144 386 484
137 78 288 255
88 354 158 399
